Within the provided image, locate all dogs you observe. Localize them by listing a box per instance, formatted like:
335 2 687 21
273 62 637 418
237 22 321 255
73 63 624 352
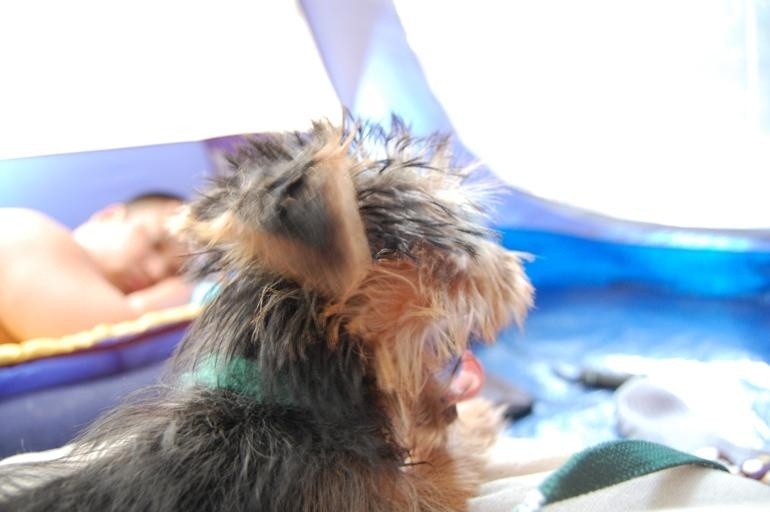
0 105 537 510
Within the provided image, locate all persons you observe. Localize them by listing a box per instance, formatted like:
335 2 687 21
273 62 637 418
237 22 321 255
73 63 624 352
0 192 196 342
